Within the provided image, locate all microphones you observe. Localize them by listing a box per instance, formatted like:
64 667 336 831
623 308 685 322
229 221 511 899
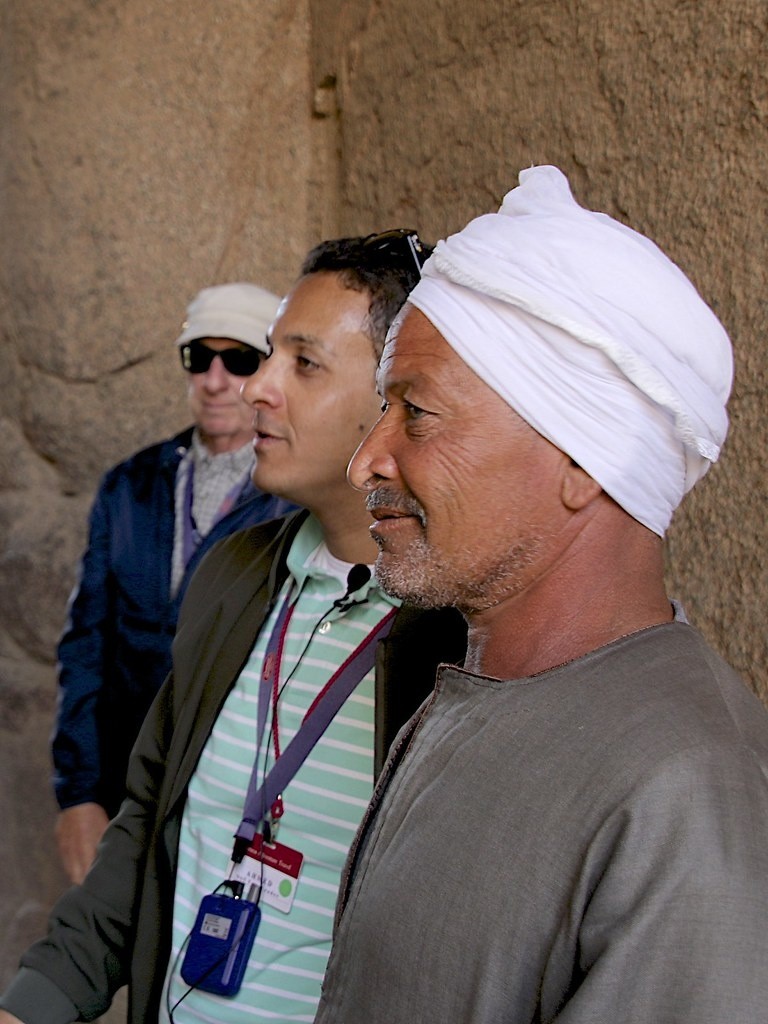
347 564 371 594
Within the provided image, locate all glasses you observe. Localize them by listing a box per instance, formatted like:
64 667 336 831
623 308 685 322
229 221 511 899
180 339 260 376
364 228 424 280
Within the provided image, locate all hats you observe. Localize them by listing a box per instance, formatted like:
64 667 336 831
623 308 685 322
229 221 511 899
406 165 734 541
175 283 284 355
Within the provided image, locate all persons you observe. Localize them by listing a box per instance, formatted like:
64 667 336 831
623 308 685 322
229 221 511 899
313 165 768 1024
0 229 469 1024
49 284 283 883
